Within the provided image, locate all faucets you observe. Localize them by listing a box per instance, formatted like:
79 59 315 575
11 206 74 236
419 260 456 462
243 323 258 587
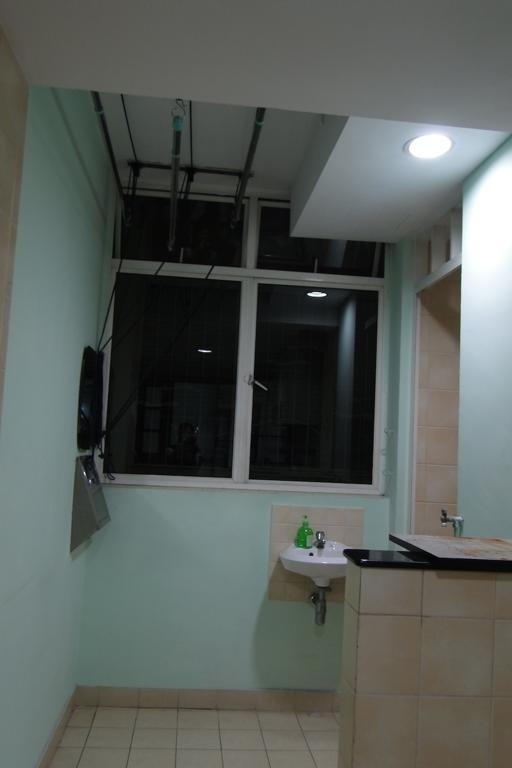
313 531 326 548
439 509 464 538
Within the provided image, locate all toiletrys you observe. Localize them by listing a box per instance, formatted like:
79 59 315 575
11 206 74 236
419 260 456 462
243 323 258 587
296 515 314 549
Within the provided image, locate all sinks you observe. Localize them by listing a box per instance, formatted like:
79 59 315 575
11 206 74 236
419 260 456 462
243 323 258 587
279 540 353 587
